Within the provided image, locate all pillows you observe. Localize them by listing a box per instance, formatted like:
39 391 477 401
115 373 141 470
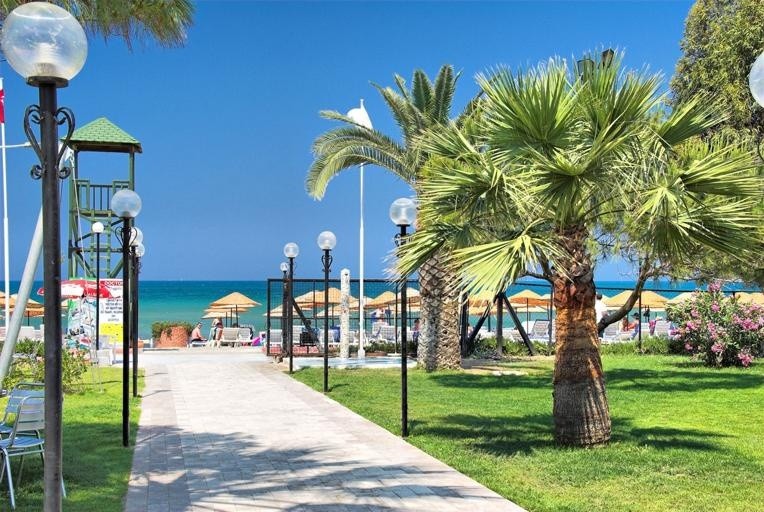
0 382 67 510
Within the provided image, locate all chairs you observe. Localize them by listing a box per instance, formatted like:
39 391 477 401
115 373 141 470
603 321 672 343
0 324 45 344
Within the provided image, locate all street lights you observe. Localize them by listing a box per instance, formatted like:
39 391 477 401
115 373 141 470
281 244 299 373
315 229 336 393
745 47 764 164
86 217 106 353
389 197 416 438
0 1 90 512
111 184 144 446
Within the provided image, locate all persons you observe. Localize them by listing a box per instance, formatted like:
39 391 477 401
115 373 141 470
190 322 207 343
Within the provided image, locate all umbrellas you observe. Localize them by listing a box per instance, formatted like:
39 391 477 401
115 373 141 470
0 291 44 326
35 278 112 336
